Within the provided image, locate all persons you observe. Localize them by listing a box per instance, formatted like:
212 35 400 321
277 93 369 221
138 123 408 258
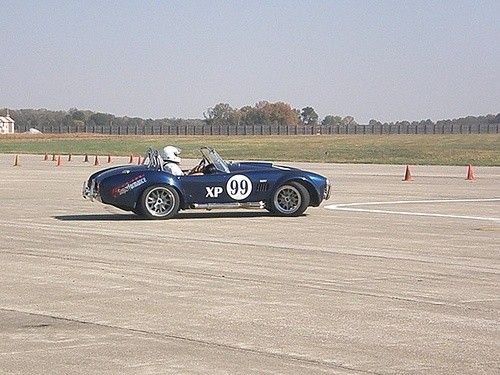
162 146 184 175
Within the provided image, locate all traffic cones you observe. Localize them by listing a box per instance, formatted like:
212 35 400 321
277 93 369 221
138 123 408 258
13 151 143 167
465 164 476 180
402 165 415 181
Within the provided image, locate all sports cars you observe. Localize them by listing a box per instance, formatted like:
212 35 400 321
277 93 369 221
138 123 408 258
81 144 332 221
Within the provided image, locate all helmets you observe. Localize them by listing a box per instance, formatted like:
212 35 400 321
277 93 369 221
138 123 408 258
164 145 182 163
